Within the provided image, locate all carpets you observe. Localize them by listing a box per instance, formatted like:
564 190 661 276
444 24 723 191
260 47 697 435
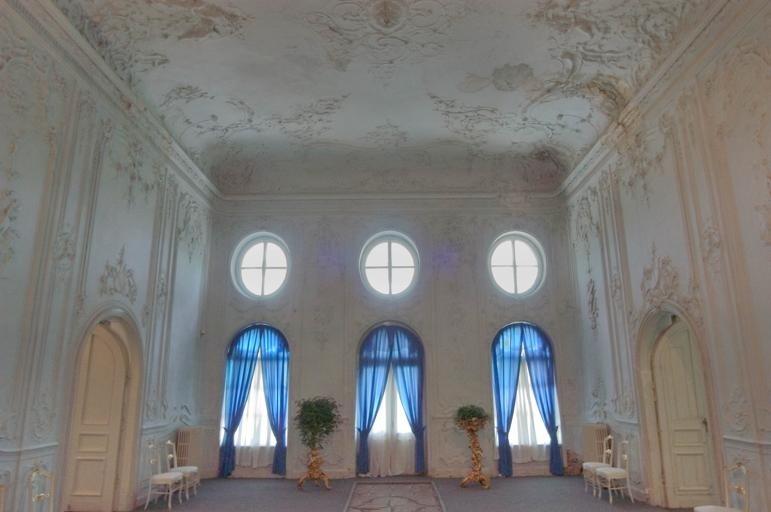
341 480 446 512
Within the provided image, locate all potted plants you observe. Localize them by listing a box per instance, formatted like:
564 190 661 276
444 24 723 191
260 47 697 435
454 403 489 489
294 393 338 489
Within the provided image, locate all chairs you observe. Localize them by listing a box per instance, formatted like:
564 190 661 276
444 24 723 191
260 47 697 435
144 444 184 511
160 440 197 501
596 440 634 505
582 434 614 497
